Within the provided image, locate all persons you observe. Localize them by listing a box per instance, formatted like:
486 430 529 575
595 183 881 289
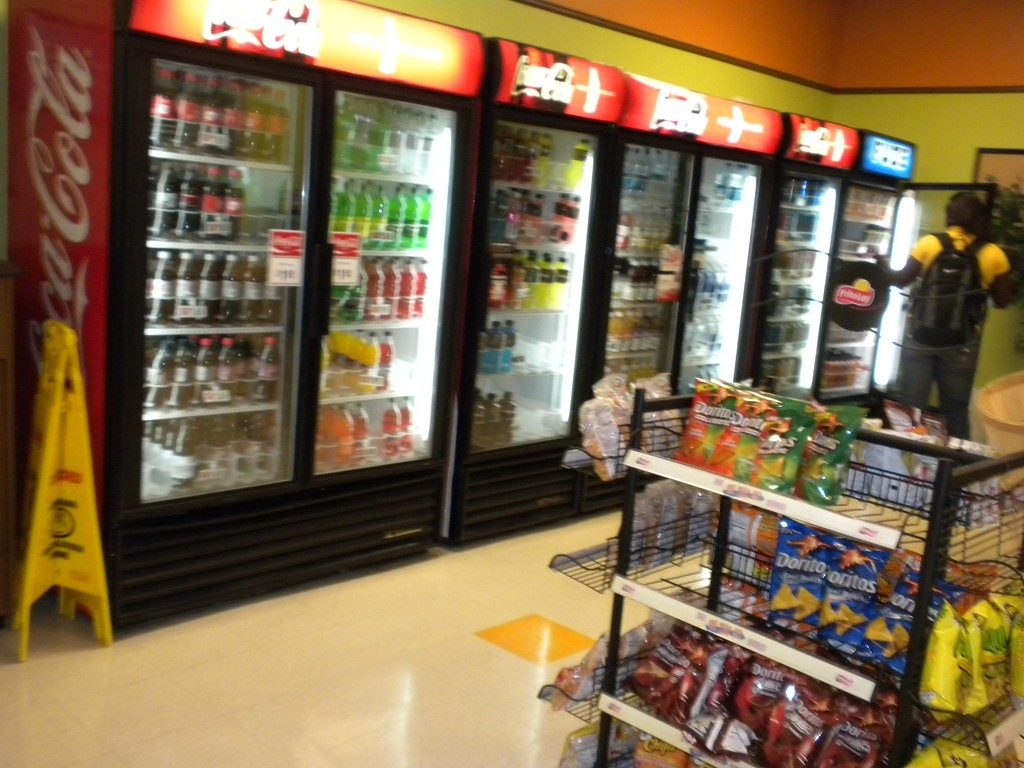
871 190 1013 443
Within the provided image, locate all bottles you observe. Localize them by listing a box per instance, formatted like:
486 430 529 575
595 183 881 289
606 143 680 386
141 52 290 488
472 121 594 450
314 97 437 469
685 162 749 396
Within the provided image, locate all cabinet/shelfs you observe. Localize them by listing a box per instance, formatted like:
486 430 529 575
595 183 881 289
534 389 1024 768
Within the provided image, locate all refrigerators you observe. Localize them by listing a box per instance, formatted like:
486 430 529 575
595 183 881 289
587 58 783 414
746 111 859 409
817 130 915 403
444 34 621 547
1 1 486 639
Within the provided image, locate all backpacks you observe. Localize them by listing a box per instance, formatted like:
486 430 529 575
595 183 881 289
910 233 987 345
